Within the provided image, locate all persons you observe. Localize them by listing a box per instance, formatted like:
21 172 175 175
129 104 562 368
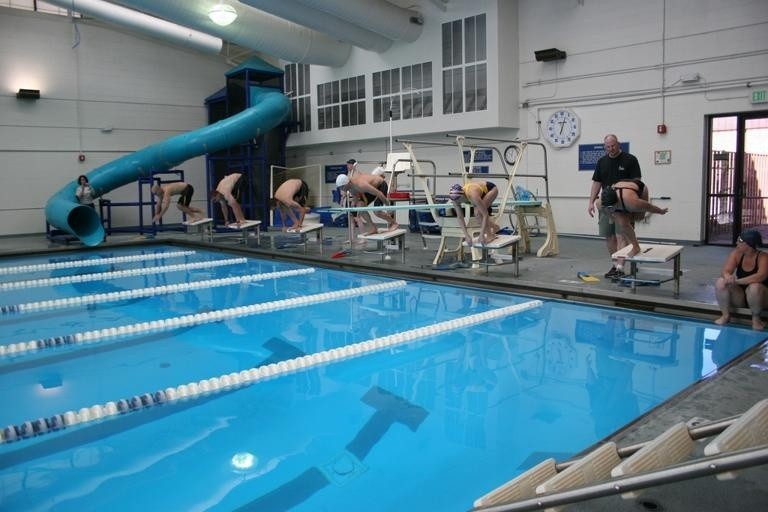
75 175 108 243
266 178 309 235
581 315 644 438
149 183 206 224
342 159 369 244
447 180 500 248
588 134 642 280
335 172 399 236
600 178 669 259
217 273 255 335
208 172 249 228
714 228 768 331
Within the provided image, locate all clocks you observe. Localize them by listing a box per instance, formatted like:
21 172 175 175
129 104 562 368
504 145 522 165
542 109 581 148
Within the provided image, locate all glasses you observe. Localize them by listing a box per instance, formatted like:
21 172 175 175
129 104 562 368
601 205 613 211
736 238 743 243
450 196 460 200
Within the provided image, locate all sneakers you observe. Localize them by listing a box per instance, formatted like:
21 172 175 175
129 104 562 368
611 271 624 279
605 267 615 278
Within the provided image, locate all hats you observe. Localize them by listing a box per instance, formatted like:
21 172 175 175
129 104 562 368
450 184 463 196
739 230 761 249
601 188 617 206
335 174 349 187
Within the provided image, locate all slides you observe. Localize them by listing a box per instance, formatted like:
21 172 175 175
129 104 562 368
45 87 292 247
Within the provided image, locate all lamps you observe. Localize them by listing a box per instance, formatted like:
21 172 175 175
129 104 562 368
533 48 566 62
15 89 40 99
